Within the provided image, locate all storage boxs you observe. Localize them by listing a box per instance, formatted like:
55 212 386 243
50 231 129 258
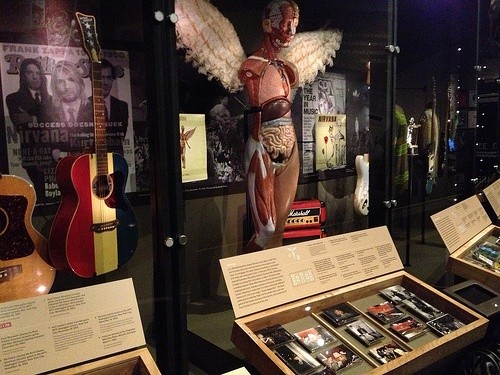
430 195 500 292
219 226 490 375
0 278 162 375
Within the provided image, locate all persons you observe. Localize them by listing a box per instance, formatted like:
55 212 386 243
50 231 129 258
208 94 232 125
173 0 342 253
6 58 128 199
180 125 198 169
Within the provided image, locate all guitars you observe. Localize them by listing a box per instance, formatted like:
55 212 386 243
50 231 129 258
1 68 56 304
47 12 139 279
